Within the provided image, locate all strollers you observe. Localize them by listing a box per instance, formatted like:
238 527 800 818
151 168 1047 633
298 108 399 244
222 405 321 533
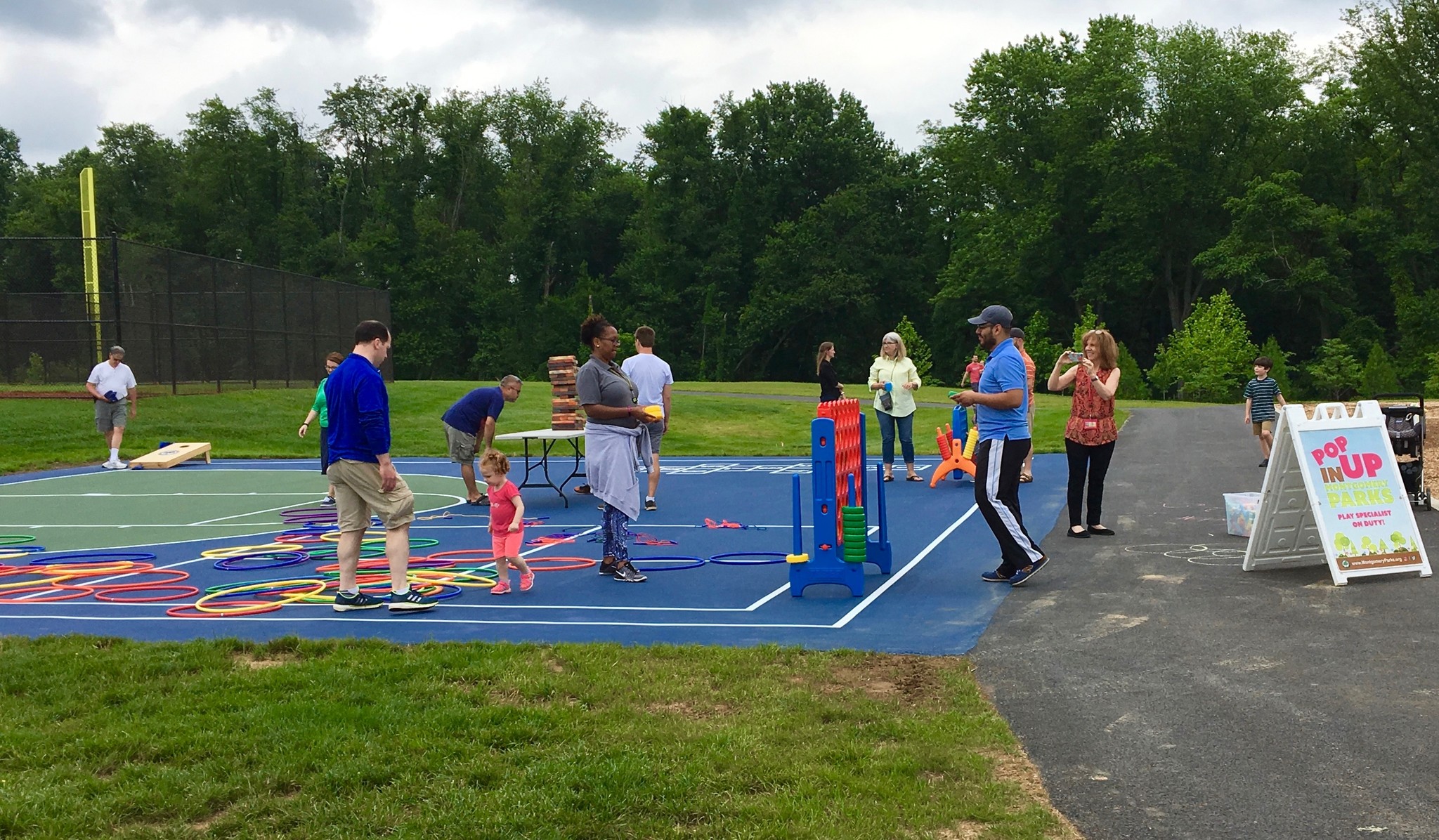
1371 392 1431 511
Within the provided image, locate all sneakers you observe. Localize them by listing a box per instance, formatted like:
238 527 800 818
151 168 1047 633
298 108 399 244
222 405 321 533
320 496 336 506
491 579 511 594
614 561 647 582
599 559 618 575
645 500 657 510
102 461 109 468
333 586 384 612
1009 554 1050 585
597 504 603 510
107 459 128 469
388 584 439 612
520 568 534 591
981 570 1010 581
574 483 591 494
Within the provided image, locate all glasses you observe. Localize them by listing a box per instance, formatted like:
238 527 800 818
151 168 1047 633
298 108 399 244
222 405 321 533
111 354 122 362
977 324 995 328
508 385 520 394
882 342 898 346
1088 330 1104 335
594 337 620 345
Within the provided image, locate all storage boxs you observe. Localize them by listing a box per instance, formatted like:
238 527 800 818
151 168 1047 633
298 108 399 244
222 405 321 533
1222 492 1261 537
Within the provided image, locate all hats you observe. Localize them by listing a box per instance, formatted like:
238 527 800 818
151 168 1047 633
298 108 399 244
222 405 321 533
967 305 1012 328
1010 328 1024 340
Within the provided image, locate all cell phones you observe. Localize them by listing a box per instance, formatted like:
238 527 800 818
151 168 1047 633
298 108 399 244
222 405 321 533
1068 352 1083 362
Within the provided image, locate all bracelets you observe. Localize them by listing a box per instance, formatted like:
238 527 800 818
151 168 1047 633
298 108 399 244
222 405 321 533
628 405 632 417
302 423 309 427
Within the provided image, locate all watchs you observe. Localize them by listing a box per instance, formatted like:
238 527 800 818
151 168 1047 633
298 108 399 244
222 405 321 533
1091 376 1099 381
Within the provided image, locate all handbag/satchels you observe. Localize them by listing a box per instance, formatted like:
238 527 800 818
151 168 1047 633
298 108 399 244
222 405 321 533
880 393 892 410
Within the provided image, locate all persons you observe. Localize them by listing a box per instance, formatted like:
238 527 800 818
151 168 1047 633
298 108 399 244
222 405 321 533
949 305 1050 586
867 332 924 482
297 352 345 507
979 360 985 378
576 313 655 584
598 325 674 511
330 318 439 613
85 345 139 470
1245 356 1286 467
817 341 846 402
1010 328 1035 483
441 375 523 506
478 448 535 594
1048 328 1121 538
961 354 984 393
573 483 590 495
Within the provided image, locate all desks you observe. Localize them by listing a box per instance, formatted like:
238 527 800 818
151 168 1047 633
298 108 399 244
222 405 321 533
495 427 586 508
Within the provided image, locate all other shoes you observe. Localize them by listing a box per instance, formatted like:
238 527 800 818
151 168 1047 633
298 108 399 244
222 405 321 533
1259 459 1268 467
1087 525 1115 535
1067 528 1090 538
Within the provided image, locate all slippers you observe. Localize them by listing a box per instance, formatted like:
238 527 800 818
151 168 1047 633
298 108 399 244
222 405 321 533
466 493 484 504
906 476 924 481
470 495 490 506
1019 473 1033 482
883 476 893 482
